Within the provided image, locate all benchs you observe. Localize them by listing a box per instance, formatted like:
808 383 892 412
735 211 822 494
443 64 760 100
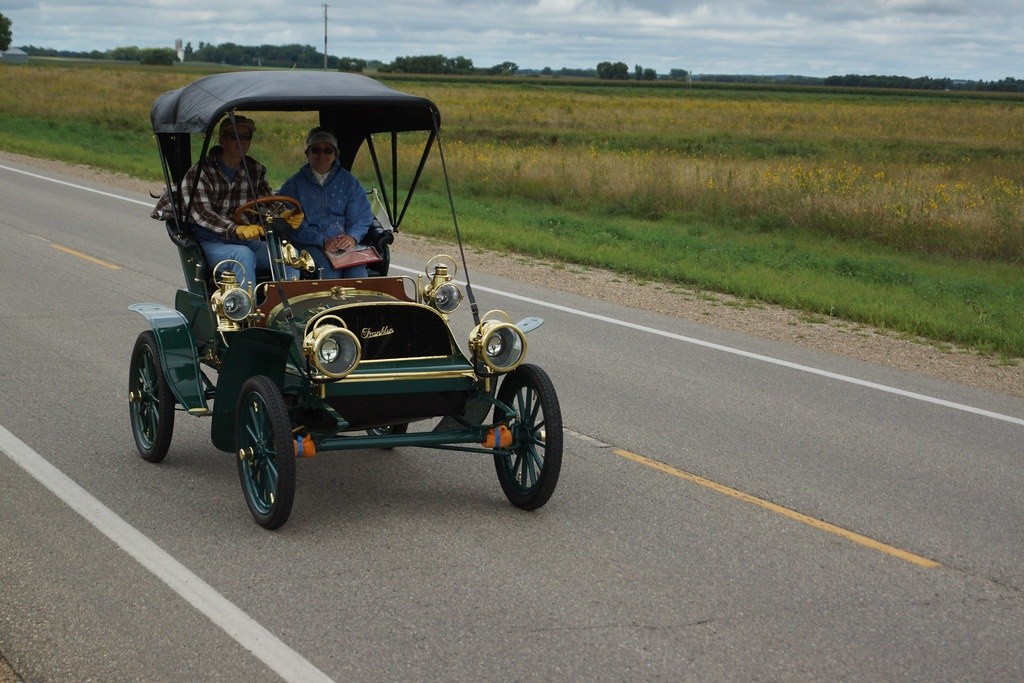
165 215 394 294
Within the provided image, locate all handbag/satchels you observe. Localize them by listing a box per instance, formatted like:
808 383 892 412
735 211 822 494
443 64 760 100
325 245 380 269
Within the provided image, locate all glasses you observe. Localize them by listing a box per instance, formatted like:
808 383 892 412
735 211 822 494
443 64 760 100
223 132 252 141
308 146 335 155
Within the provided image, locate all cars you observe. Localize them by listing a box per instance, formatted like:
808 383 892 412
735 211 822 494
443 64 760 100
129 72 564 529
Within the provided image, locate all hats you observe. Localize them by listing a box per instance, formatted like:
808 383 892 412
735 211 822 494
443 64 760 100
219 116 256 136
304 127 340 156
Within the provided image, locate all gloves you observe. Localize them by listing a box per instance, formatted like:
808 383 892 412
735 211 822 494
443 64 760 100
236 224 265 241
279 209 304 229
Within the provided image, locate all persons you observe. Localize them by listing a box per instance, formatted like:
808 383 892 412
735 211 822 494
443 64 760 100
272 126 373 280
181 115 304 331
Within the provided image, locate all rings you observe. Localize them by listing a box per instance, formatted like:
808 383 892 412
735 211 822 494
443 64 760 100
348 242 351 244
